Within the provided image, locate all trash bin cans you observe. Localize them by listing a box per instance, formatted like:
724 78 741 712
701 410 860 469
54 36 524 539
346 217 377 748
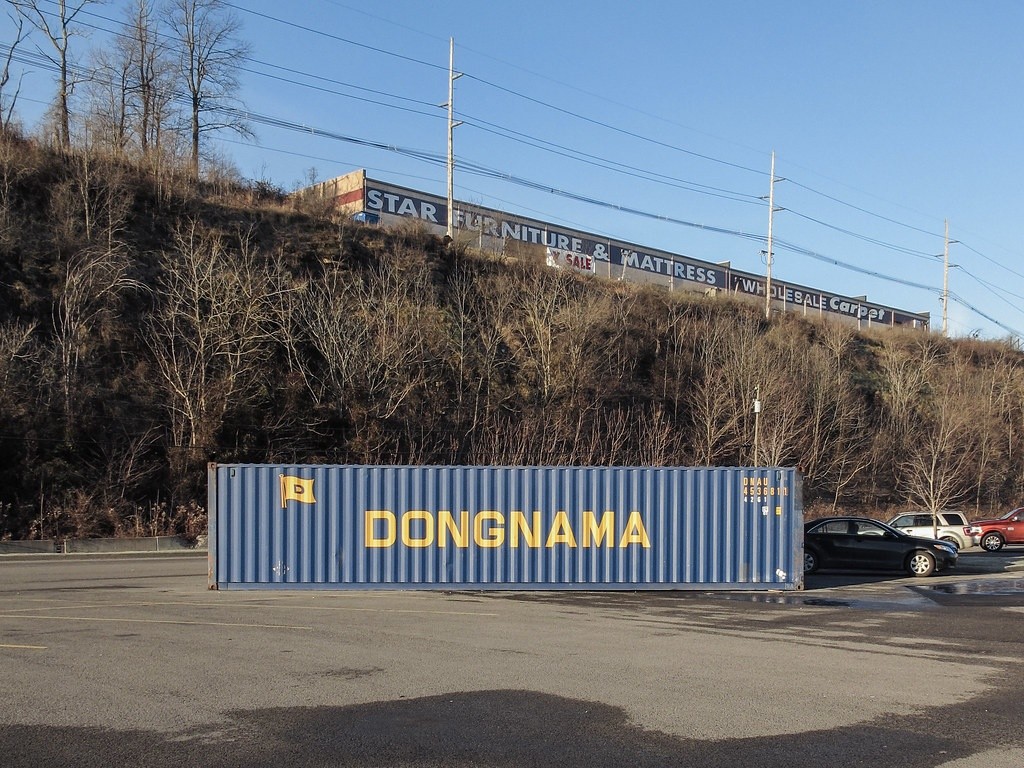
350 211 379 224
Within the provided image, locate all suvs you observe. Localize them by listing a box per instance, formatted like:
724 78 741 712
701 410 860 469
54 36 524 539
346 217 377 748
857 510 975 552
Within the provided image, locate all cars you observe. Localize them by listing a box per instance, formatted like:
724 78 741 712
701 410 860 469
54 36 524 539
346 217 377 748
804 516 960 577
971 507 1024 552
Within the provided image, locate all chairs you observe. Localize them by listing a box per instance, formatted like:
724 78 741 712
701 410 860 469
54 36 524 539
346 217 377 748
855 524 859 535
